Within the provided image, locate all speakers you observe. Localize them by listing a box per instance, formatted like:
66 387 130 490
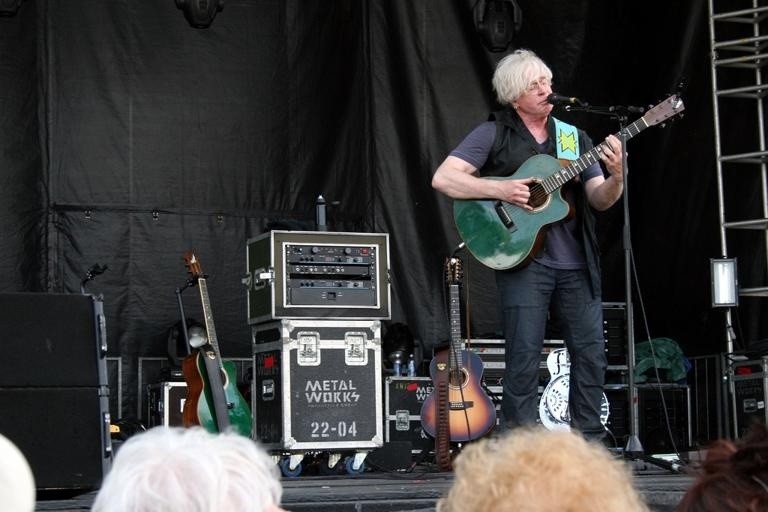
0 387 114 490
0 291 108 387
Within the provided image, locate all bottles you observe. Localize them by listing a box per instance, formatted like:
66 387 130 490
407 353 417 377
393 353 403 376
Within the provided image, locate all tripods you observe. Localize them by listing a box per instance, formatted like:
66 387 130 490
577 104 687 474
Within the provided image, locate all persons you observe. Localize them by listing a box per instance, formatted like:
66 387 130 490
90 425 291 512
434 420 652 512
673 420 768 512
0 434 37 512
431 47 631 446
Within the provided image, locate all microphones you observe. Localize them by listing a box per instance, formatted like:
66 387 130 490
548 92 576 105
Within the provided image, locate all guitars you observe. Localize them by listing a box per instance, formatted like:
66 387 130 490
182 251 256 435
538 341 611 436
419 256 496 443
453 93 685 271
